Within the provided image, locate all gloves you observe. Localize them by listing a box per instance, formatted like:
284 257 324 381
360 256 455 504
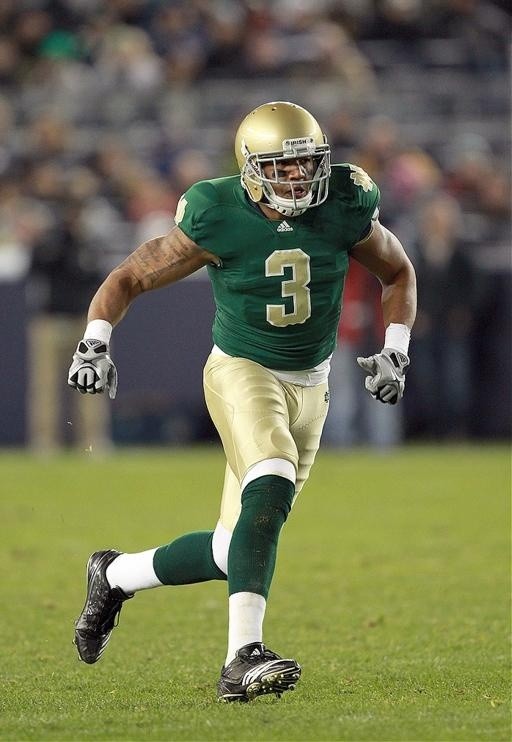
66 338 119 400
357 347 411 404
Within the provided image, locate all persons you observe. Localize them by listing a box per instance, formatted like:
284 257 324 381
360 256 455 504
1 1 512 457
67 100 419 704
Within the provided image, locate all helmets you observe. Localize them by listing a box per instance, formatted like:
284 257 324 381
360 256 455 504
234 101 333 217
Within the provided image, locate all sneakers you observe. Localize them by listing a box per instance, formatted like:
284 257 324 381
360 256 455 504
217 641 302 704
73 549 135 665
